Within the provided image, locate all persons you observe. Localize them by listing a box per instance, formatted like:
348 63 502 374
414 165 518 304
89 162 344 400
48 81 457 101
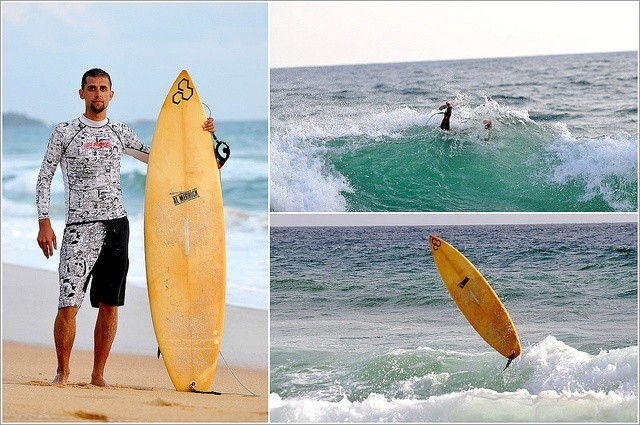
36 68 216 387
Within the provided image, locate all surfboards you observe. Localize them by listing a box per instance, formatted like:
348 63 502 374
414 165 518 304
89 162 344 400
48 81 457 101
144 70 226 392
429 234 521 360
